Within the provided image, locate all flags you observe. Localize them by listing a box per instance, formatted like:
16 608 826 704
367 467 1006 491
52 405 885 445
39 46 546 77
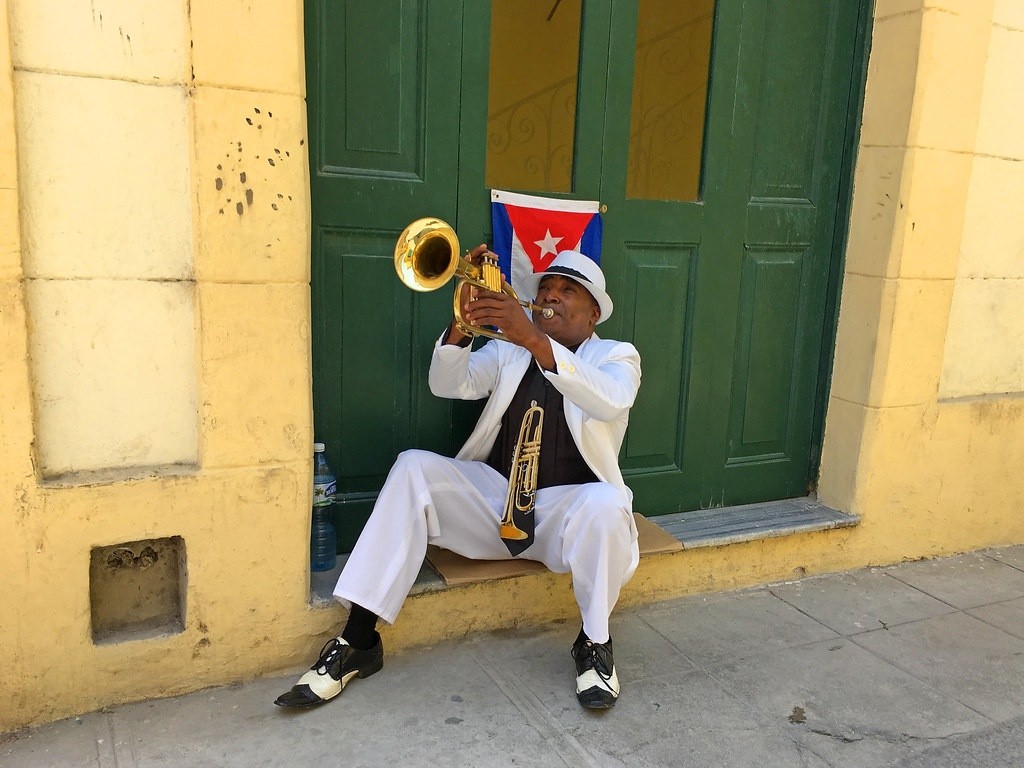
491 188 604 333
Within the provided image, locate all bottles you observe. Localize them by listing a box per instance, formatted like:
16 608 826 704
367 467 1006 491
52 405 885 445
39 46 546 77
309 438 337 573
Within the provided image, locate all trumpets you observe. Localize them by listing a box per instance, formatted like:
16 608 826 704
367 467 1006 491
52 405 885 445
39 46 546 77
394 218 554 345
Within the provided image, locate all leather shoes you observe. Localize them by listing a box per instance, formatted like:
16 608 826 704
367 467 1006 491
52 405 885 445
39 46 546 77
273 630 384 710
573 621 621 709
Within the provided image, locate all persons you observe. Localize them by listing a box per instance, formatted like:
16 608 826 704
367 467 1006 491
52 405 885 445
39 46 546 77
273 251 642 709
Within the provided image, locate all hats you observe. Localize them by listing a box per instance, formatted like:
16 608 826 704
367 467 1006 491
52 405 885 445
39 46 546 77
524 250 614 325
